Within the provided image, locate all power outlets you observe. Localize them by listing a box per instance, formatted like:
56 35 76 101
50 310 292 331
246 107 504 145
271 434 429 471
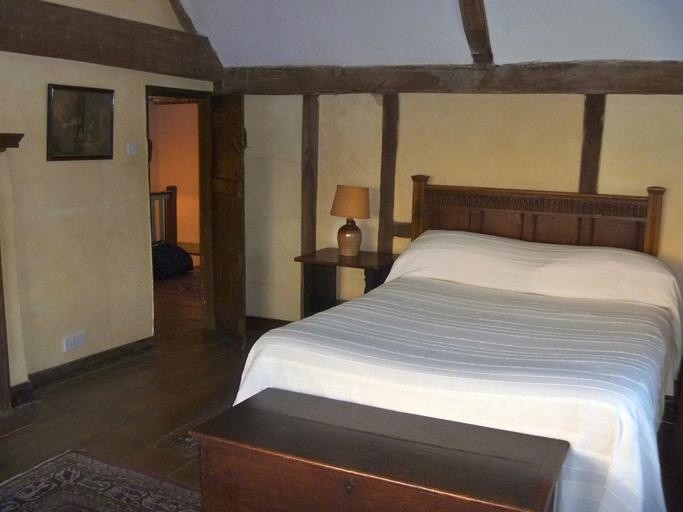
63 335 88 352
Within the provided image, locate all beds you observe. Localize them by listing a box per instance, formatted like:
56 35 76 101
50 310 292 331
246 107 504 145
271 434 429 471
235 175 681 512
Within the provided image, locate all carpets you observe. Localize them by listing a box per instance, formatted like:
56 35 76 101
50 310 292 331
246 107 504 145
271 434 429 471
0 448 199 510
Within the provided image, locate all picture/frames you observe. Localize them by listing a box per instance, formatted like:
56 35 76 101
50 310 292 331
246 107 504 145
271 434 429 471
45 83 115 162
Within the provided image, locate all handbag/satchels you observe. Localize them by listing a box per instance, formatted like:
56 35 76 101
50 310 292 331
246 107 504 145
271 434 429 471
152 244 193 279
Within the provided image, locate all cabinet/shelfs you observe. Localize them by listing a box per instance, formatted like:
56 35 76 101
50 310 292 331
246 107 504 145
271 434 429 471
293 246 401 319
187 385 569 510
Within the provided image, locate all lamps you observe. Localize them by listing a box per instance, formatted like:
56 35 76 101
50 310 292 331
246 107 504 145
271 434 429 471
329 184 369 256
